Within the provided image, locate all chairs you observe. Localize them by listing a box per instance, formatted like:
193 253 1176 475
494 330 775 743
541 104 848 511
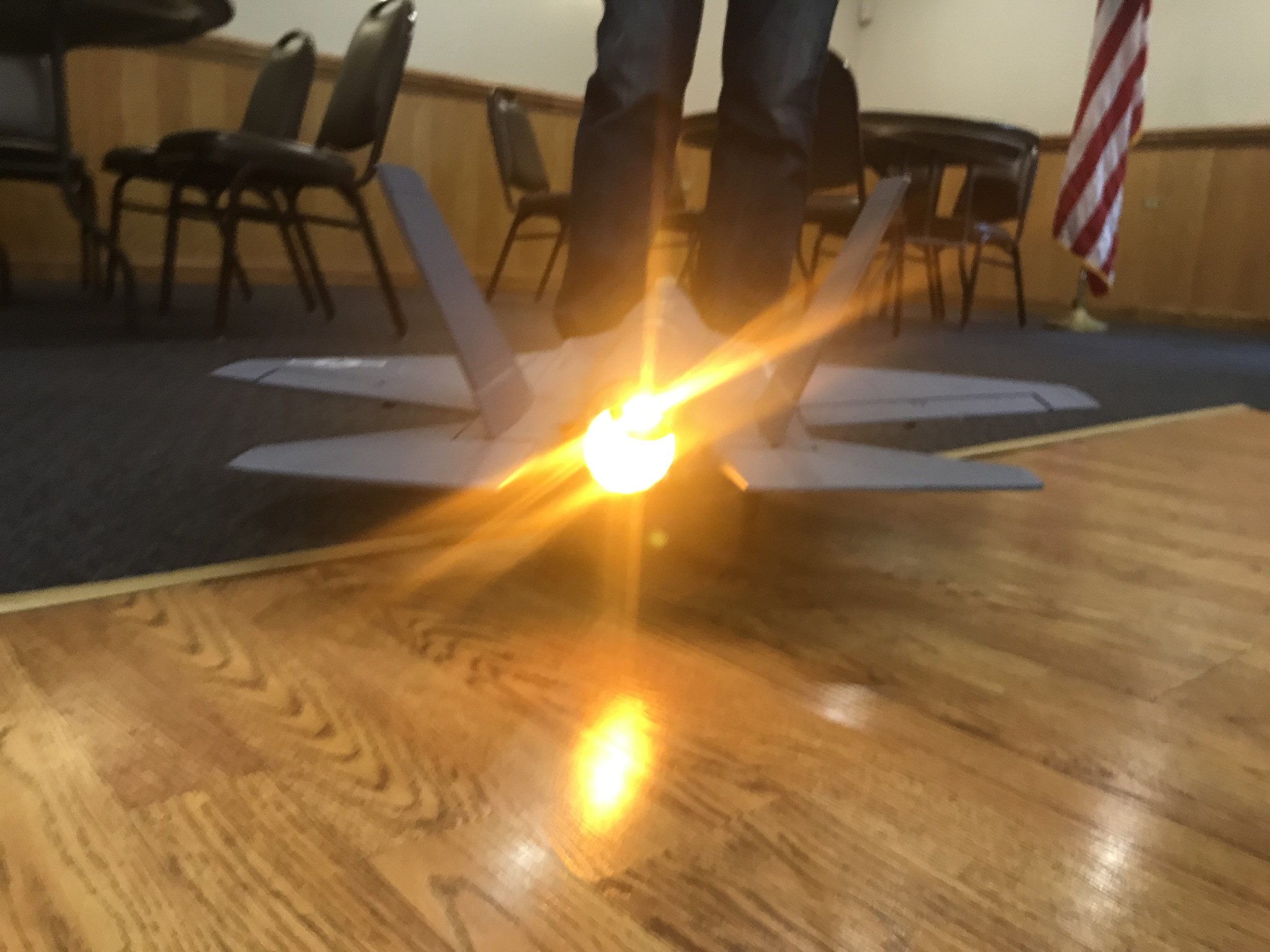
662 137 1037 336
485 88 574 302
102 0 422 341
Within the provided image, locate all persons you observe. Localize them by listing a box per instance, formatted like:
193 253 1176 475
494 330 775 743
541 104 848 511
551 1 841 365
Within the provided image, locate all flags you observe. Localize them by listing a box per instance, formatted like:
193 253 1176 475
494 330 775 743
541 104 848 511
1050 0 1151 295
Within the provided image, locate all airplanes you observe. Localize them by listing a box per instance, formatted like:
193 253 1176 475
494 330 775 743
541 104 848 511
212 163 1103 564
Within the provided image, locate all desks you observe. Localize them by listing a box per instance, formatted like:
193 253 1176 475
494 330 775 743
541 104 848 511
687 112 1039 337
0 0 242 343
